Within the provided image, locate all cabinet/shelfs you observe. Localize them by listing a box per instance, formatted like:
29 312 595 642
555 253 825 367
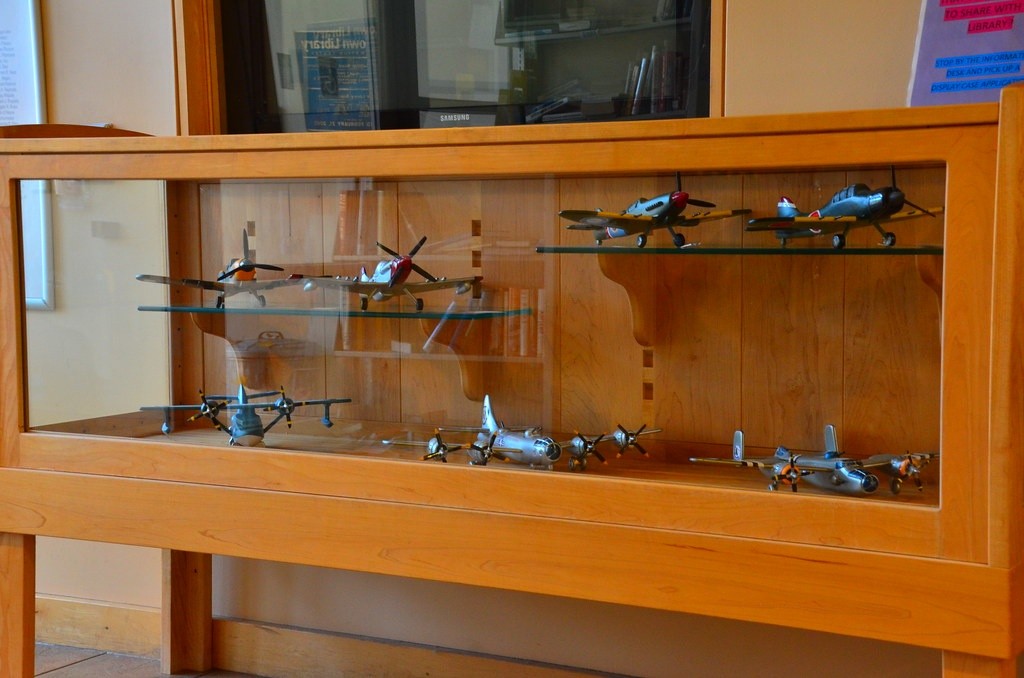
0 78 1022 677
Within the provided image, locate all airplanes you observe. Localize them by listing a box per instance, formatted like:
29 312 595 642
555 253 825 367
686 422 942 505
131 228 307 307
381 395 669 471
139 383 355 452
552 188 752 248
742 181 946 254
304 237 487 311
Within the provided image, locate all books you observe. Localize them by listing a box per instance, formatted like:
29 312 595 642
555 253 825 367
504 0 685 122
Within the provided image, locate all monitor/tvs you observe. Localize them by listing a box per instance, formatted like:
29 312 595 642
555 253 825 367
245 0 709 133
